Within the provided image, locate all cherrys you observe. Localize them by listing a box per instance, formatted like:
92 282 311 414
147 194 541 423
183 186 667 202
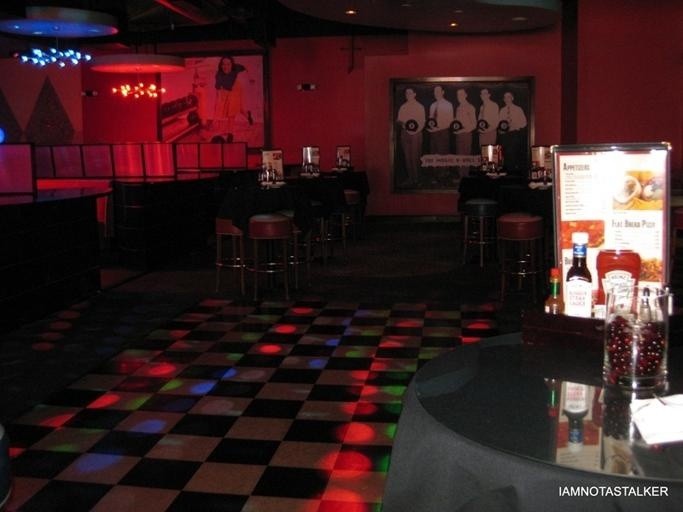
607 315 668 386
601 387 669 453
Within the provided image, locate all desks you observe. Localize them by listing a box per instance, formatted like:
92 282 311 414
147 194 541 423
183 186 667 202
388 326 681 510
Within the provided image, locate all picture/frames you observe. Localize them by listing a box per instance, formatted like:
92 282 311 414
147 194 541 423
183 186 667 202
157 49 270 154
387 74 534 195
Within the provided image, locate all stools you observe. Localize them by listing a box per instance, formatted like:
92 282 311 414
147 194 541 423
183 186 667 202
463 198 547 304
215 188 360 304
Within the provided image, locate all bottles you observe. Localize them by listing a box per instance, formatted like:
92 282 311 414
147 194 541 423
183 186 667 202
564 232 594 317
563 381 591 456
544 267 563 315
545 377 562 417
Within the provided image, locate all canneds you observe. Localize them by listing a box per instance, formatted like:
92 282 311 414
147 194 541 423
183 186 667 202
596 247 639 317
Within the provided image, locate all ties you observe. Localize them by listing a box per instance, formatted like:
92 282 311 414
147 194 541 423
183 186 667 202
433 104 438 121
506 107 513 124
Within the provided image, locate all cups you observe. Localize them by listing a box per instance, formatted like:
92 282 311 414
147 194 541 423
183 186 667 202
602 286 670 396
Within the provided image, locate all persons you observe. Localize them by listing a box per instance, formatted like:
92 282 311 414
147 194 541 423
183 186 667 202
212 57 248 119
397 86 527 183
